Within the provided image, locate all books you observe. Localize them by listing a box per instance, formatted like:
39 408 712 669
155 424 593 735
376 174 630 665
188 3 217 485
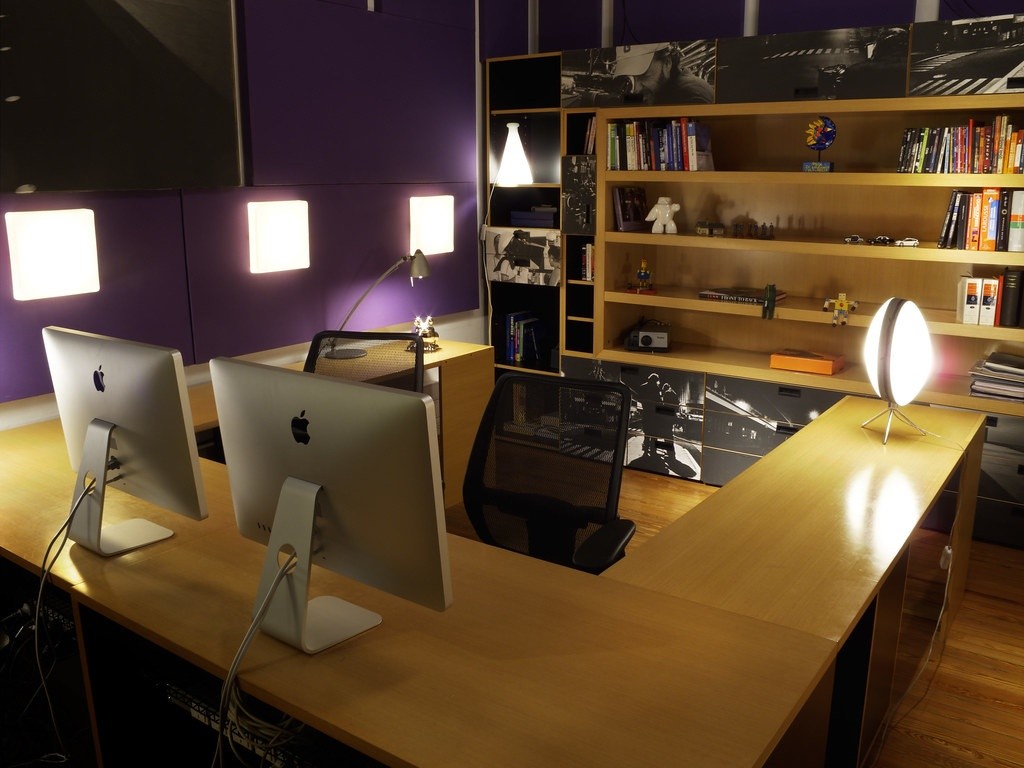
956 267 1024 329
897 114 1024 174
938 189 1024 253
613 185 651 231
582 117 715 171
967 351 1024 403
582 244 594 282
505 311 543 363
699 286 787 304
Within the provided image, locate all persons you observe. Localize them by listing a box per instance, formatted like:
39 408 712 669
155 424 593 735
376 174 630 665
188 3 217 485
613 43 714 106
822 293 860 327
492 230 560 287
640 373 679 449
733 222 774 237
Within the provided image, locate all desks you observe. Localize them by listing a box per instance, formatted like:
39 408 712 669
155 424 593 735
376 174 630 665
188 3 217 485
0 334 989 768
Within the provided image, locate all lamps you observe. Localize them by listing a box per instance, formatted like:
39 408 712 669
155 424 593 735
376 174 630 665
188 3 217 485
323 251 430 361
860 298 947 439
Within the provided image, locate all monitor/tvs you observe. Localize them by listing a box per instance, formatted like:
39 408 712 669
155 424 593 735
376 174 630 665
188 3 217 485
41 325 209 556
209 355 453 654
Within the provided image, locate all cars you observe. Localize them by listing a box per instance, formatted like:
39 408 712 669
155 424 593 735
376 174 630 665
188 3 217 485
894 237 920 247
844 235 864 243
867 236 895 246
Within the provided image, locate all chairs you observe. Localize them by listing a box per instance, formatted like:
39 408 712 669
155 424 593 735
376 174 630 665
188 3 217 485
464 372 633 575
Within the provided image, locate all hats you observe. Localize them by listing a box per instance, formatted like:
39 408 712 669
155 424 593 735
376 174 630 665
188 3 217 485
612 42 672 80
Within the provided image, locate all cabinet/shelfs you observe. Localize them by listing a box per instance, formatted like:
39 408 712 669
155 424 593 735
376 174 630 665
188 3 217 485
486 16 1024 549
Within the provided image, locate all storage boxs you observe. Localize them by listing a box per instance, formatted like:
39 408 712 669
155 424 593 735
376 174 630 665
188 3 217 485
769 348 845 375
